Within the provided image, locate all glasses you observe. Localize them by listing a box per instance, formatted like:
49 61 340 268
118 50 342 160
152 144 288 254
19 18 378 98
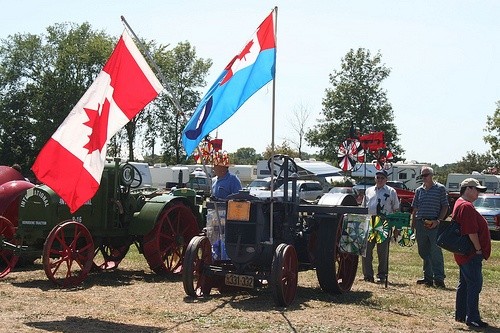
421 173 431 178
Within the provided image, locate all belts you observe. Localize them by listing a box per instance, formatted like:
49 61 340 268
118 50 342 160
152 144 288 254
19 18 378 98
416 218 438 222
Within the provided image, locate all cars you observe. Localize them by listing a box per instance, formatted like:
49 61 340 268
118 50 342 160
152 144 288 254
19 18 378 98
247 178 324 202
328 186 363 205
470 193 500 235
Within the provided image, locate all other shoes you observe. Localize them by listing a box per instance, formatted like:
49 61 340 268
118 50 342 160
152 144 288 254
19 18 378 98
467 321 489 328
377 278 386 284
417 280 433 286
434 280 445 289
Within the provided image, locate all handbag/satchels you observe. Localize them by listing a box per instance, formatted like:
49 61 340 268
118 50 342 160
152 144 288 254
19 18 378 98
436 219 474 256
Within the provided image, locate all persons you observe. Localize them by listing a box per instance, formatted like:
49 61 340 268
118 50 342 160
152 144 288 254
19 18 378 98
206 155 242 260
12 164 43 185
409 165 450 290
437 178 491 328
359 169 400 283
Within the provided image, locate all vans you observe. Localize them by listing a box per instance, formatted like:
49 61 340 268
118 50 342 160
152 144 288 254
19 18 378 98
445 169 500 195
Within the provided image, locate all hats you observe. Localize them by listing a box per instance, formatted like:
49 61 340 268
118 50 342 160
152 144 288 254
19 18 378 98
460 177 487 193
375 169 387 178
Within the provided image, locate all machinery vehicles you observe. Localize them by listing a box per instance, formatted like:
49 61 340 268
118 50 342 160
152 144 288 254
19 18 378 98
0 151 204 290
181 153 369 308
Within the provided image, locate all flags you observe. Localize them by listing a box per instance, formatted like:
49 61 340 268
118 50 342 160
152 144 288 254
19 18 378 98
31 28 164 214
182 6 278 159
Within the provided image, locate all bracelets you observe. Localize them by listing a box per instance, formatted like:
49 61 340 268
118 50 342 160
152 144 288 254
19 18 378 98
476 248 482 251
437 218 443 224
